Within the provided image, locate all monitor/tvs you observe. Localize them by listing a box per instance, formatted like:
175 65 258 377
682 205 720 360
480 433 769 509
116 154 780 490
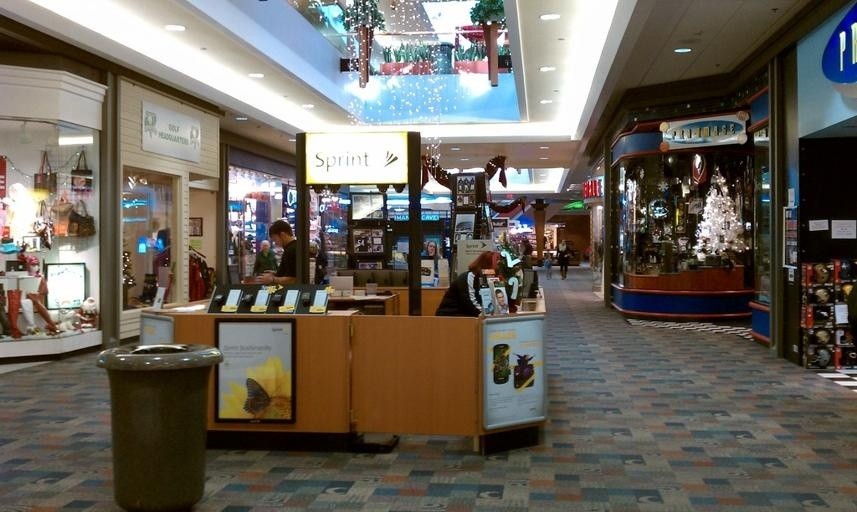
43 262 87 310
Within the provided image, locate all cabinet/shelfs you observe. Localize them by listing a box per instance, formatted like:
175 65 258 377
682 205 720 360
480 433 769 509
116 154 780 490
328 293 400 315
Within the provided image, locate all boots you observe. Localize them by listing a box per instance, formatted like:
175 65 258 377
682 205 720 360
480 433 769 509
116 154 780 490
1 289 59 338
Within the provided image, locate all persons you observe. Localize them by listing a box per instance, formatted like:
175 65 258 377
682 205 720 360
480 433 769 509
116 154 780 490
434 251 498 320
73 297 97 328
228 230 347 276
356 230 437 275
513 238 534 270
555 238 572 280
544 255 553 280
246 218 296 284
496 288 508 314
27 256 57 330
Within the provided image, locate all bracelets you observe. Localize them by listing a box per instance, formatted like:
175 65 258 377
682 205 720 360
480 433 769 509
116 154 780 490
271 277 275 283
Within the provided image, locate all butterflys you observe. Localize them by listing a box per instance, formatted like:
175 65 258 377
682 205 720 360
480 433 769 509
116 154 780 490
242 378 272 419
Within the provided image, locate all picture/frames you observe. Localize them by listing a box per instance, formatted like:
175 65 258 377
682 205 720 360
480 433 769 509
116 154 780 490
190 217 203 236
214 317 297 424
482 313 547 432
138 312 175 345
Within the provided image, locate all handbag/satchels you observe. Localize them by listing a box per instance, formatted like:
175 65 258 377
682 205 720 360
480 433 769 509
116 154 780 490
34 150 96 250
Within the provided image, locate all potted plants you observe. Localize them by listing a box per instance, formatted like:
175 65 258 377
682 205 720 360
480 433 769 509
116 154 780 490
380 42 511 74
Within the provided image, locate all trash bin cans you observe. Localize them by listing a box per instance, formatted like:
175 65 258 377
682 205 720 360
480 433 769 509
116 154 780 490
97 344 224 511
